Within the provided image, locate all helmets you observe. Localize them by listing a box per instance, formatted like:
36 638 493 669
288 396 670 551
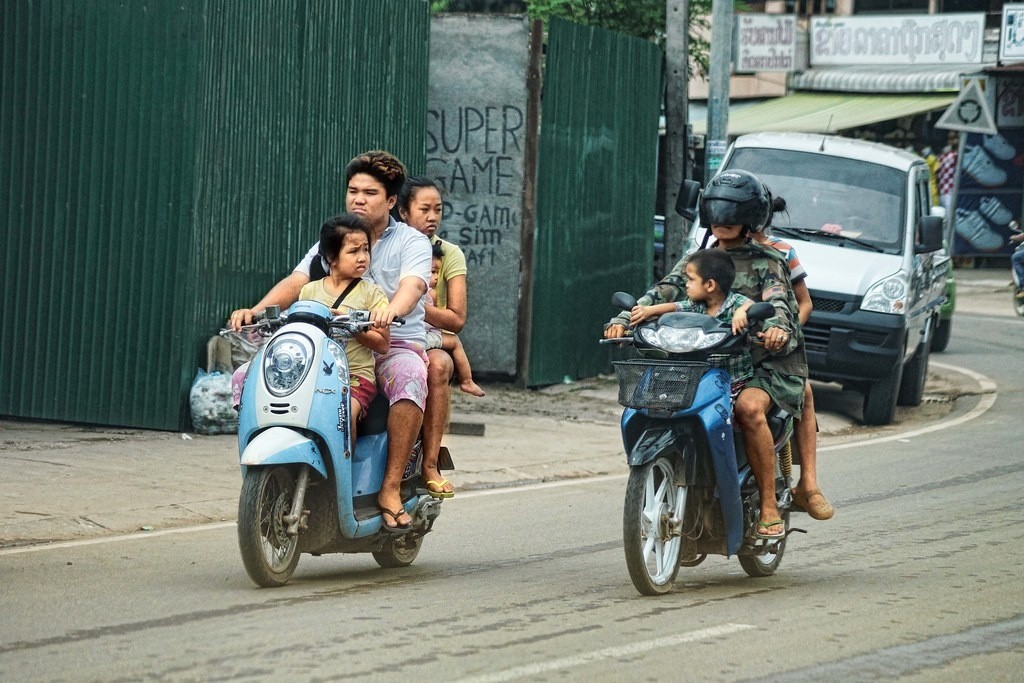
699 168 770 234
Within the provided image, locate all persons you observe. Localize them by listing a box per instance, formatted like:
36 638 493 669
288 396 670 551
605 167 834 538
1010 232 1024 299
225 147 486 530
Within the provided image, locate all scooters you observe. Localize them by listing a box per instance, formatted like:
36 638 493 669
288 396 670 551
222 305 454 589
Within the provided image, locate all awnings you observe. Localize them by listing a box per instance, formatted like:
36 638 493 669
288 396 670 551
787 61 996 89
652 92 960 138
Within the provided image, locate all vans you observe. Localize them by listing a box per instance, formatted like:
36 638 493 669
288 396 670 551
673 133 951 428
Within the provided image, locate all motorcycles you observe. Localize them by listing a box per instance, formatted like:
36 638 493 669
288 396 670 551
1009 221 1024 315
597 291 812 598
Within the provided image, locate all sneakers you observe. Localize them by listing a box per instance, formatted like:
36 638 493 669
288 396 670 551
961 144 1008 188
978 195 1013 226
955 207 1005 252
983 129 1017 161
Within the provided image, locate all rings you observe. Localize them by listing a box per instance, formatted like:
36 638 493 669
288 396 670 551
777 335 782 339
782 337 788 340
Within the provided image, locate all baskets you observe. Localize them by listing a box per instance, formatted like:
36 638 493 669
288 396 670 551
611 357 712 410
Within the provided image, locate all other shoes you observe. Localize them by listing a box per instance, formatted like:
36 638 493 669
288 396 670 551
1015 290 1024 299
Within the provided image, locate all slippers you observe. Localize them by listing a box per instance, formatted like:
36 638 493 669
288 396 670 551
790 485 835 520
756 519 787 540
381 507 414 534
426 478 455 499
